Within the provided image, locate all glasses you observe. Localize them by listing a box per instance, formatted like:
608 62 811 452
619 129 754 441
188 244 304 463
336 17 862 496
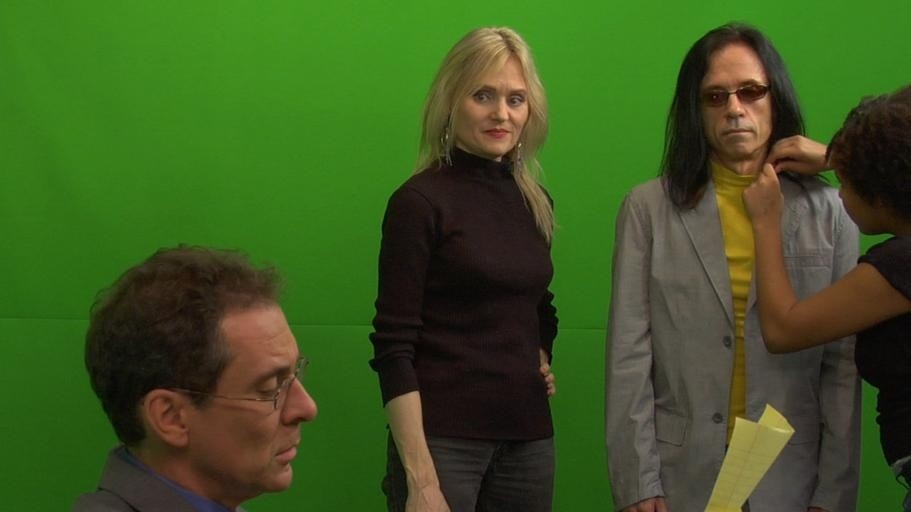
699 84 770 107
174 355 310 411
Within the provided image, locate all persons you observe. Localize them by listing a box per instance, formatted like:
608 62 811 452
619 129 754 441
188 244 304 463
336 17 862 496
369 26 561 512
741 82 910 512
71 241 318 512
603 18 863 511
